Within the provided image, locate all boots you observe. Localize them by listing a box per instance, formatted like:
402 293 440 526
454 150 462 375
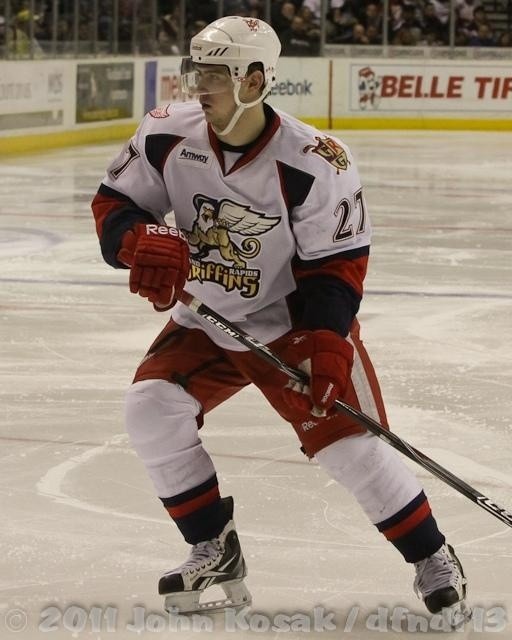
404 531 468 616
156 497 248 597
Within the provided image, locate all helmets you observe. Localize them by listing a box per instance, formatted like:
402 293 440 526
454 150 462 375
181 13 283 110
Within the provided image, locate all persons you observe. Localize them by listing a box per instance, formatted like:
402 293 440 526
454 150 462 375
90 14 468 614
0 0 512 62
